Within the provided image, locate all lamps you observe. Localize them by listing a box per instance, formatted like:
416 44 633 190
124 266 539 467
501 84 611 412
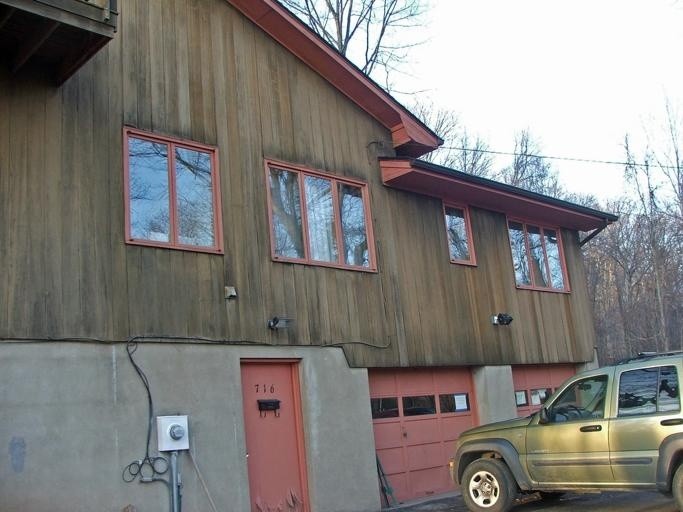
494 314 514 327
270 315 295 328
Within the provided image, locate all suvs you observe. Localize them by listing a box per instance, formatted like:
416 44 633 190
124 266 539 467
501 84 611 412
449 351 683 511
